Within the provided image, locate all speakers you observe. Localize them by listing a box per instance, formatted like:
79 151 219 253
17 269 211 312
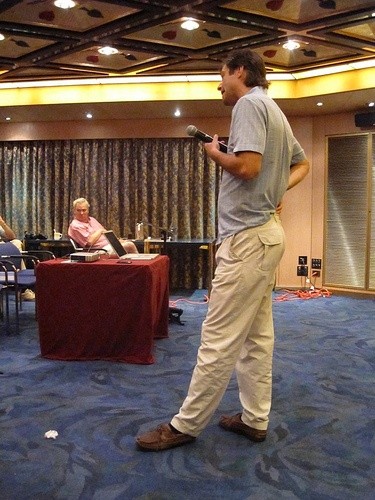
355 111 375 126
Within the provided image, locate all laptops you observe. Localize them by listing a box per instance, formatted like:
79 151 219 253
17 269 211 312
102 230 159 260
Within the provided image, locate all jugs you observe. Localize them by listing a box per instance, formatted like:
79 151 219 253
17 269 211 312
135 223 144 240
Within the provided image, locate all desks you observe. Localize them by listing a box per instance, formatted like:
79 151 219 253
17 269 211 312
24 237 216 363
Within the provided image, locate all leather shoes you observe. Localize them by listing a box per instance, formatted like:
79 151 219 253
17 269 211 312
136 423 196 450
219 413 267 442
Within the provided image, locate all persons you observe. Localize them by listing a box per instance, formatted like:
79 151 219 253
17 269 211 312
137 47 310 449
68 198 139 259
0 216 36 300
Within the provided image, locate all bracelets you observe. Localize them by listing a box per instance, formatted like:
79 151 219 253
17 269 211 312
1 223 6 229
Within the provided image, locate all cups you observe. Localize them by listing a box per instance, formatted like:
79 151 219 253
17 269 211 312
54 232 62 240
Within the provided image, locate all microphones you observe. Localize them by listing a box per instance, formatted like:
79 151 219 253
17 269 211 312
186 125 227 153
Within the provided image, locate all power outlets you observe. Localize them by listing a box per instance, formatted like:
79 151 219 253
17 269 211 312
299 256 307 264
313 270 320 277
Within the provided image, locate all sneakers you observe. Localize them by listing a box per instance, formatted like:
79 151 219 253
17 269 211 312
18 288 35 301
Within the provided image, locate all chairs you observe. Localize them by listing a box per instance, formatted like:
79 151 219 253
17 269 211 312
0 238 108 334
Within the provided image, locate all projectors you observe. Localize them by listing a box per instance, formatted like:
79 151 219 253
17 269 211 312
70 252 100 262
